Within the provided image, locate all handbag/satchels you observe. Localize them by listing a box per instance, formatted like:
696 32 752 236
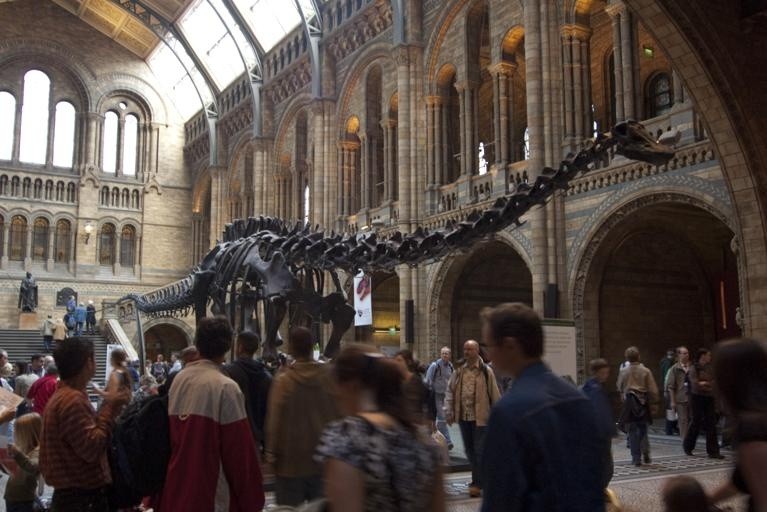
114 393 171 497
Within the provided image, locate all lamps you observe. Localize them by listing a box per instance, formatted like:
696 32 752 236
83 225 92 244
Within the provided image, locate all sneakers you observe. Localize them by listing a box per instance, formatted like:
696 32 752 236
470 487 480 496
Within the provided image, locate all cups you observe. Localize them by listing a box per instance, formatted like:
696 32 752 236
387 326 396 336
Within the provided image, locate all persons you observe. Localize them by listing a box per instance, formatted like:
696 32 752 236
578 359 622 510
444 338 502 496
16 272 38 312
1 314 455 511
40 295 96 352
613 338 765 510
472 300 610 510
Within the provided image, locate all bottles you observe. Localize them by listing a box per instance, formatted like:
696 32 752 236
682 382 689 402
91 382 99 390
312 337 320 361
5 424 16 460
